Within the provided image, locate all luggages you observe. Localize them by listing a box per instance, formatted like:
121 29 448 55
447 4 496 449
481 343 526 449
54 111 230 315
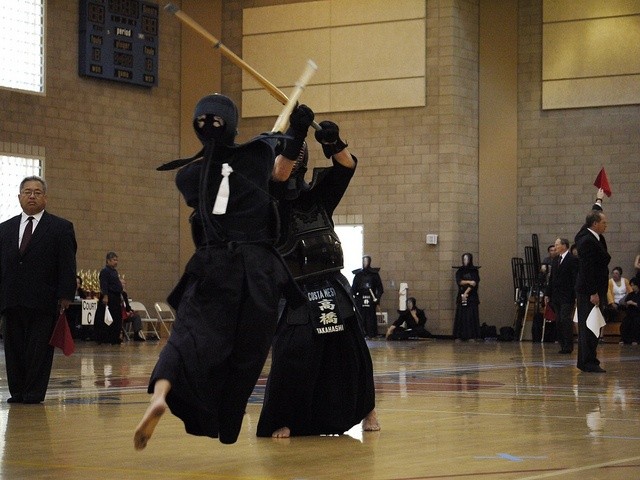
532 279 556 342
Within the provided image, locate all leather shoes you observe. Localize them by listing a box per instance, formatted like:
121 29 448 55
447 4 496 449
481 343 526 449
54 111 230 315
578 360 606 372
7 394 23 403
23 396 41 404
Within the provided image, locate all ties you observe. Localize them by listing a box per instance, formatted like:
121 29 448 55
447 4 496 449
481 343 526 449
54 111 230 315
19 217 34 257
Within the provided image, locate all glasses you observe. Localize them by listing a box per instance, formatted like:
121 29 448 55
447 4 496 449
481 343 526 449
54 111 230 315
21 192 44 197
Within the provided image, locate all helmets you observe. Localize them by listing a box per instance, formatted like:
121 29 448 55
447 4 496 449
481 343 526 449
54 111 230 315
276 136 309 177
192 94 238 145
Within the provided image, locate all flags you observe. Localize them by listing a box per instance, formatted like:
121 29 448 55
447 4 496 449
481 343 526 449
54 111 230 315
104 306 113 326
544 303 555 320
593 167 612 198
586 304 607 338
49 312 76 356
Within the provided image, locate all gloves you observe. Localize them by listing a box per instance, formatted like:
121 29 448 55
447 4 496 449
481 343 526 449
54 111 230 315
280 101 314 161
315 121 348 159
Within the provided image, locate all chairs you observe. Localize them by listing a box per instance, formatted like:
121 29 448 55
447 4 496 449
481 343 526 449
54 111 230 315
122 300 161 341
152 301 178 338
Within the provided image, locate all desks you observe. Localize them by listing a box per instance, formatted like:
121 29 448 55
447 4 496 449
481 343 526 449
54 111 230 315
60 301 88 342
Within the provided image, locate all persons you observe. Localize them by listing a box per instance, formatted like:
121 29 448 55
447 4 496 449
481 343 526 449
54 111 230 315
570 244 578 256
99 252 124 345
618 277 640 346
607 266 634 322
544 237 579 354
634 255 640 279
385 297 433 340
121 291 146 342
0 176 78 404
453 252 481 344
132 93 310 450
254 100 382 438
574 187 611 373
74 275 86 302
538 245 556 281
351 256 384 340
